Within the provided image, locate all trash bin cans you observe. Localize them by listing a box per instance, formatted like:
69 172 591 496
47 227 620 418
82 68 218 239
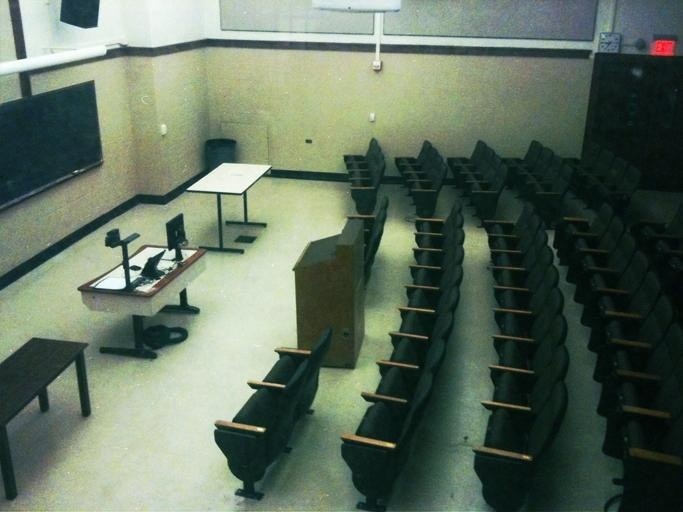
205 138 236 172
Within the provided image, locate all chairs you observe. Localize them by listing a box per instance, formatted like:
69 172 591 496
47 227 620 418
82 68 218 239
212 326 336 502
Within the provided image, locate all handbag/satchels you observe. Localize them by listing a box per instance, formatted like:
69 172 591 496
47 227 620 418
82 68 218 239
143 325 187 348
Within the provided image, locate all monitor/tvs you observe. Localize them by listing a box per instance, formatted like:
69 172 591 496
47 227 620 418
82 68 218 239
166 213 188 261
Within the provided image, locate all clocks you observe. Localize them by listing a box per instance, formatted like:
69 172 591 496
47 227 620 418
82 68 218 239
597 32 622 56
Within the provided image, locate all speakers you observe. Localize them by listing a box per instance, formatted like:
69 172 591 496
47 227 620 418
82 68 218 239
60 0 100 28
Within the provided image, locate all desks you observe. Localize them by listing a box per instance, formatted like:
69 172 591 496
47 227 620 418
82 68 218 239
185 160 273 255
77 241 208 360
0 336 91 502
